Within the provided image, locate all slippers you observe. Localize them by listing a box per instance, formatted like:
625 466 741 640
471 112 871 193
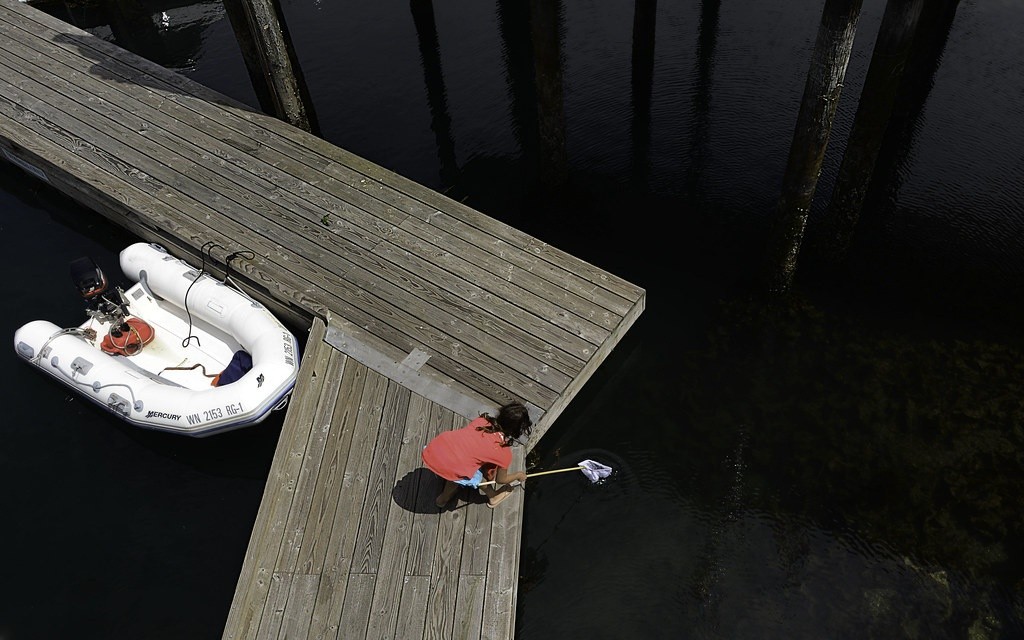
435 483 459 508
486 483 514 508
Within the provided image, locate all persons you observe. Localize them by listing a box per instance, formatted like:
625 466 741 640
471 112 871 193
421 401 532 509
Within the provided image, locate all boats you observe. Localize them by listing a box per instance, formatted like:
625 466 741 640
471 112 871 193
14 242 299 438
